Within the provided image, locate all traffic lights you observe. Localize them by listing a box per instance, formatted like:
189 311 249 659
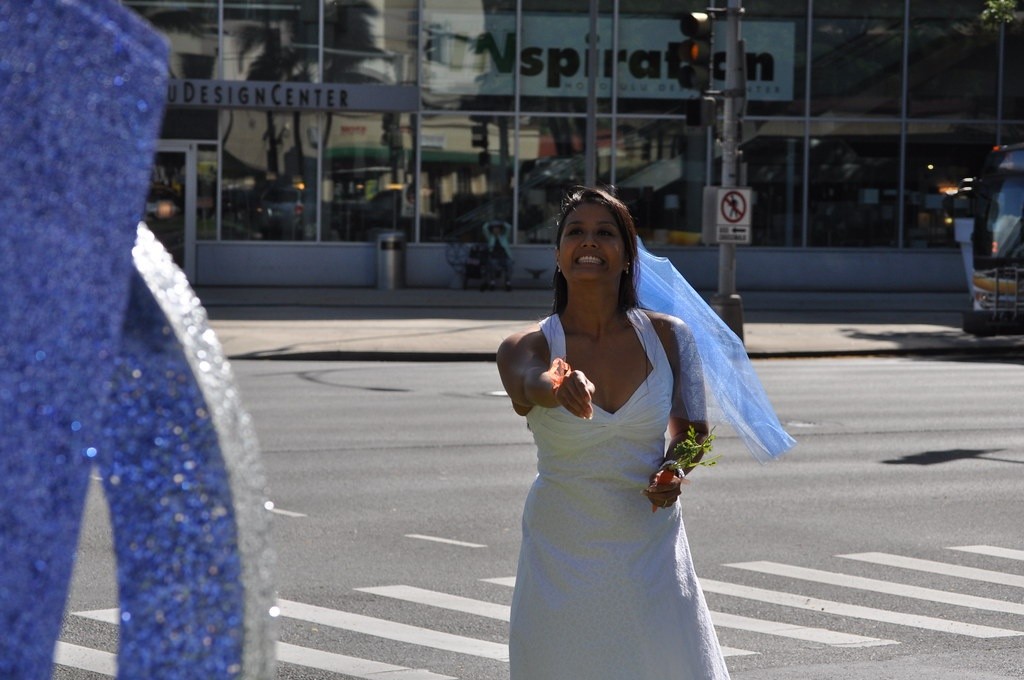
681 9 714 89
471 123 491 165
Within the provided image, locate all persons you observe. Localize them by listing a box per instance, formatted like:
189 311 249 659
482 221 514 291
496 188 730 679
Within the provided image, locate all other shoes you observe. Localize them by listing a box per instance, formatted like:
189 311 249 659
505 285 511 292
490 284 496 291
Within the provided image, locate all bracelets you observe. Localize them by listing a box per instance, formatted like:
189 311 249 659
549 357 572 404
659 460 685 478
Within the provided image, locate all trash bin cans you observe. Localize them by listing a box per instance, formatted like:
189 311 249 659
376 231 407 293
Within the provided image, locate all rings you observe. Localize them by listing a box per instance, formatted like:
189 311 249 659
663 499 667 507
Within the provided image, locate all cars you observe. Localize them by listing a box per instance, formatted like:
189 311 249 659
963 144 1024 340
524 131 963 251
241 162 424 246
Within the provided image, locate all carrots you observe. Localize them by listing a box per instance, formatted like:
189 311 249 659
652 467 675 512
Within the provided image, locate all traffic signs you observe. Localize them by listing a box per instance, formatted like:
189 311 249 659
715 187 753 248
